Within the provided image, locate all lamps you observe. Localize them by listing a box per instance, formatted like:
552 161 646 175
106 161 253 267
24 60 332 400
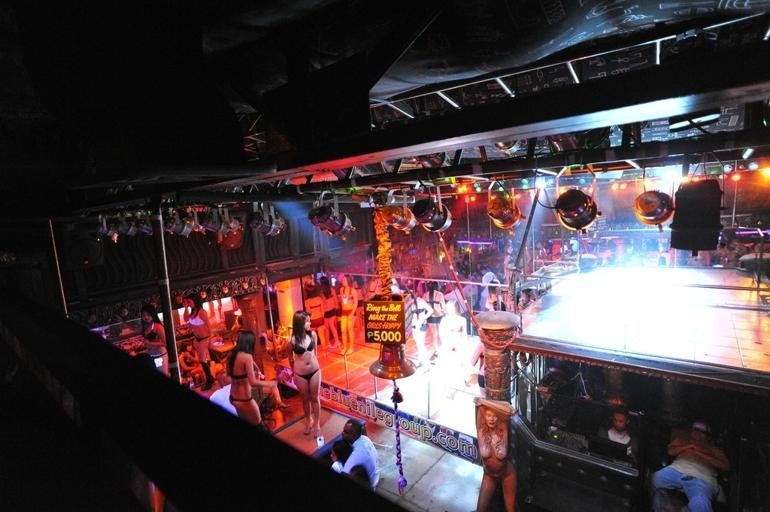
71 164 729 261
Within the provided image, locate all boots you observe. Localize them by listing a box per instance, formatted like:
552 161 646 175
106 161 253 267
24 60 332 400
200 362 213 390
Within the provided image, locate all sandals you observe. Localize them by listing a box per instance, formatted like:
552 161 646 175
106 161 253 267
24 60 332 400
314 429 321 439
304 424 312 435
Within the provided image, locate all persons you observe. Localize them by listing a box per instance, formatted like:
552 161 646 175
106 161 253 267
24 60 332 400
651 422 731 512
287 310 321 439
179 342 206 384
596 409 642 469
227 331 277 425
329 418 381 491
471 342 485 397
474 397 517 512
141 304 169 378
184 293 214 390
305 237 579 368
